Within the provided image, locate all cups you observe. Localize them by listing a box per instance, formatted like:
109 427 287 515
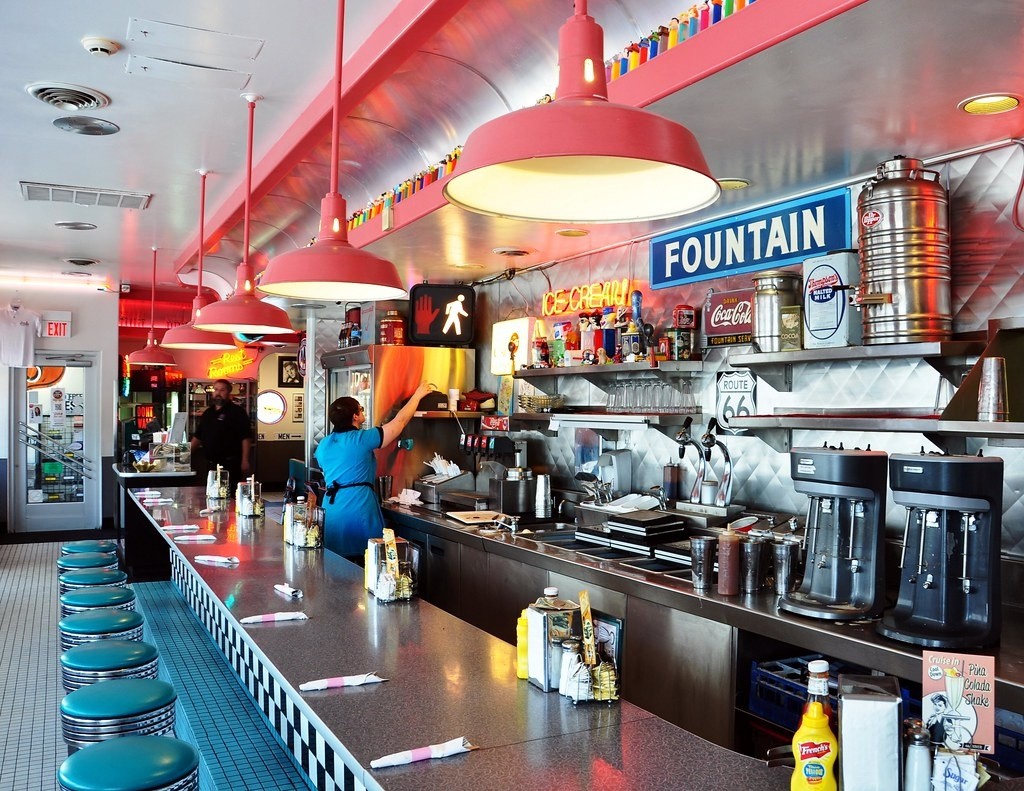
770 540 800 595
688 534 716 589
739 538 763 595
976 356 1009 422
376 476 392 504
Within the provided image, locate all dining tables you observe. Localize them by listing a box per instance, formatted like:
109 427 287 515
127 486 794 791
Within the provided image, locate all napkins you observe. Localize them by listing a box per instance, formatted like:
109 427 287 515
134 491 161 495
370 736 472 769
200 506 221 514
239 611 308 624
194 555 240 563
161 524 200 530
174 534 218 540
143 498 173 503
298 672 382 692
273 583 304 598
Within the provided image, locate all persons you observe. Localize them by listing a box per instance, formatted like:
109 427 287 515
283 361 300 383
314 380 433 561
191 379 249 500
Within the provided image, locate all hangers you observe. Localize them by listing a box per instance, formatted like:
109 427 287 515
9 292 23 311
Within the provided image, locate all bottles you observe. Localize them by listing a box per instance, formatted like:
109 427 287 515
543 586 559 601
530 316 550 367
790 702 838 791
561 640 579 695
750 271 803 353
379 311 407 346
797 660 835 736
515 609 530 679
550 637 565 689
604 378 699 414
364 550 412 600
856 155 955 346
283 496 308 547
664 327 691 360
717 523 739 595
904 733 933 791
235 477 252 514
337 322 362 349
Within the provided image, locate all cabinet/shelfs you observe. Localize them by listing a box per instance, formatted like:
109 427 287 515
510 339 1024 437
181 377 249 443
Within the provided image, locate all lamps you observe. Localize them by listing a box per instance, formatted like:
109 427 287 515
126 244 178 366
442 0 724 225
158 167 239 351
254 0 408 302
189 95 296 334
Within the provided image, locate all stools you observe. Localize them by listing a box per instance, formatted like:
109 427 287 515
57 736 200 791
56 552 119 579
59 679 178 758
59 586 136 620
60 640 159 695
59 568 128 596
61 540 116 557
58 608 144 653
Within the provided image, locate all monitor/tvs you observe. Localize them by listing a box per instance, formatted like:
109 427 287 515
130 369 165 392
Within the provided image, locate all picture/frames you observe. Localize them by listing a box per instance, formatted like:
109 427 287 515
278 356 304 388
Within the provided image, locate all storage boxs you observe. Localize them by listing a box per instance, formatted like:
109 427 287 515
837 674 902 791
207 470 227 499
285 503 314 546
748 654 908 739
237 481 257 515
525 599 579 692
367 537 408 596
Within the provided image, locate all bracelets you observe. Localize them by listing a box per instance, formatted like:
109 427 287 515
414 393 419 404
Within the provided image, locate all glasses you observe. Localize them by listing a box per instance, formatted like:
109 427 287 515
358 406 364 413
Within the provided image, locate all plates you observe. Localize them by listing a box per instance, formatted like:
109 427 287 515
517 394 560 413
446 510 514 524
